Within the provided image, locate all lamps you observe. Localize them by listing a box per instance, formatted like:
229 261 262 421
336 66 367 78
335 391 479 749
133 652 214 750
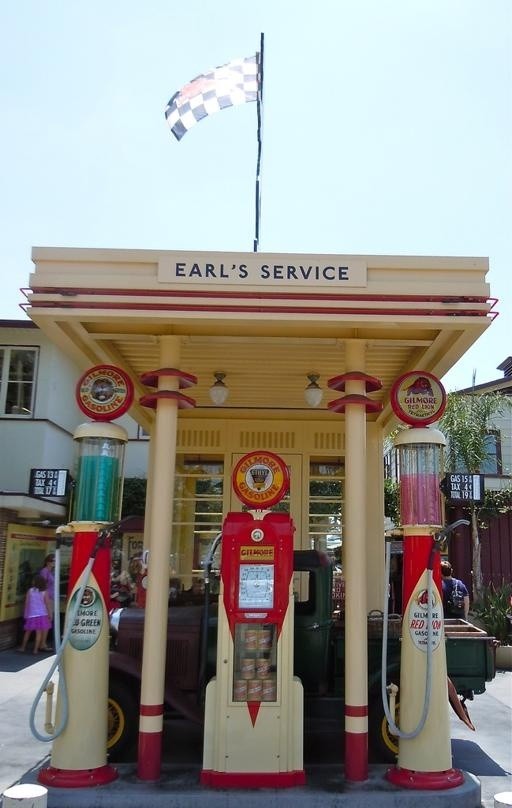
302 371 324 408
209 371 230 406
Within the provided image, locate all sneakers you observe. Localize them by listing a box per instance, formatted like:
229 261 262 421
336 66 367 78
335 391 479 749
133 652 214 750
17 648 25 653
33 651 38 654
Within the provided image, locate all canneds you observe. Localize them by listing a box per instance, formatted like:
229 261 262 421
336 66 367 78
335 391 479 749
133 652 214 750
248 679 262 701
240 657 255 679
234 679 247 701
258 628 272 650
256 657 271 679
244 628 258 650
263 680 276 701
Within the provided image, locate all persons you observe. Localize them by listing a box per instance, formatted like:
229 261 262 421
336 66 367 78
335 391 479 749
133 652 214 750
15 572 52 655
440 560 470 622
37 554 56 653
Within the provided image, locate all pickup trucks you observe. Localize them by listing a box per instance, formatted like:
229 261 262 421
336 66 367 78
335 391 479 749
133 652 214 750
107 548 497 765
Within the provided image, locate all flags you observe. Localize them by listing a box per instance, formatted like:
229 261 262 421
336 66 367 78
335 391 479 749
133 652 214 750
163 51 261 142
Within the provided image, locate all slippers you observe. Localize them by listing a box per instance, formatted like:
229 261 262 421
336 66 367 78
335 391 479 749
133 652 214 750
39 646 53 651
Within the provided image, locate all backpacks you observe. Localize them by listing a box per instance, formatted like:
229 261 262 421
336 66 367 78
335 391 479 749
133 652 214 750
448 580 464 614
